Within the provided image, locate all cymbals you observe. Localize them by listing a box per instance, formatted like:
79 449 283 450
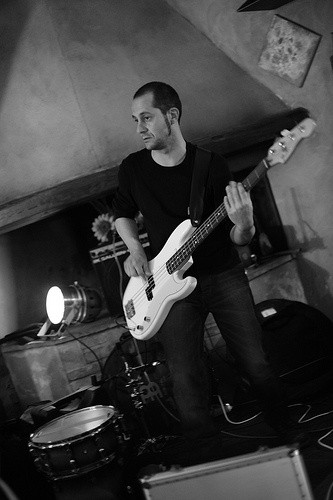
93 359 172 388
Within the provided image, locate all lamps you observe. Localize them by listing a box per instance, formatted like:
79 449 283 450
37 284 102 340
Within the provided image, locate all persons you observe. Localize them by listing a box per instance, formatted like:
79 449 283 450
114 81 281 467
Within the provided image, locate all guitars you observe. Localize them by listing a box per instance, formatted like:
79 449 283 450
122 117 318 341
218 356 329 424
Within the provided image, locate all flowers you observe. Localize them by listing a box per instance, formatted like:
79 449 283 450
92 213 115 243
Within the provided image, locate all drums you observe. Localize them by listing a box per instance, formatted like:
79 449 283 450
28 404 128 483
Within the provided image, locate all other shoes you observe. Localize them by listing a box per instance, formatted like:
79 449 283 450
275 418 311 448
